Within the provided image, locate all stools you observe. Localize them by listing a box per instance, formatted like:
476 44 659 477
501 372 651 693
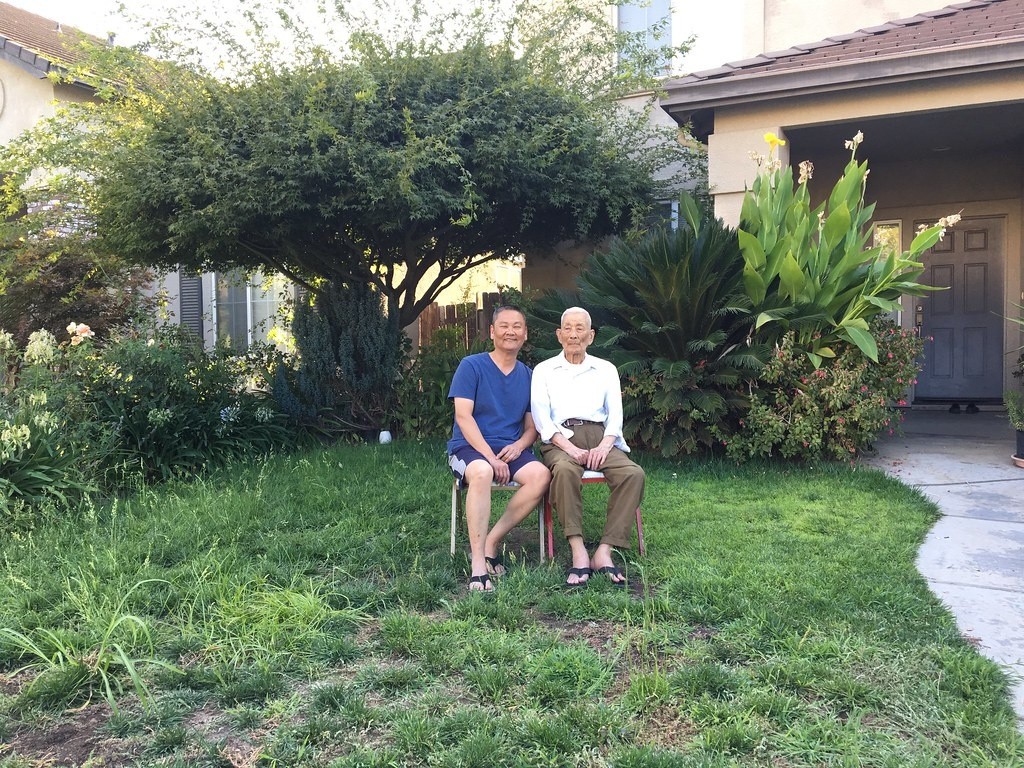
545 468 645 561
451 450 545 564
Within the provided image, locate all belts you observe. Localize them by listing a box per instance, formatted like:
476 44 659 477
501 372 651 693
561 418 603 428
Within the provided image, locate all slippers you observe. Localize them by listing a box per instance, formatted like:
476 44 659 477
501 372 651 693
468 553 506 577
591 566 630 584
566 568 592 586
469 575 495 595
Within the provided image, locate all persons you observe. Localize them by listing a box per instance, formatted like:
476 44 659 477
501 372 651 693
531 307 646 587
444 305 553 594
